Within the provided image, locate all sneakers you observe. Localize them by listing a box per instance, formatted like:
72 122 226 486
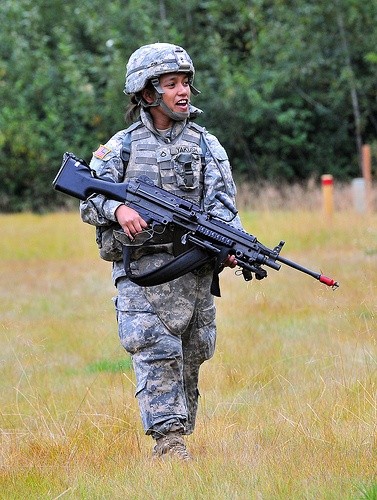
154 436 201 481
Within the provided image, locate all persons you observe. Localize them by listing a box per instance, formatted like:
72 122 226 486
80 43 244 463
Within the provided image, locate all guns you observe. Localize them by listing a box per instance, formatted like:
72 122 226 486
53 152 337 287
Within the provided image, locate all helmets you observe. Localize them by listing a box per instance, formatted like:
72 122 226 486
123 43 195 96
